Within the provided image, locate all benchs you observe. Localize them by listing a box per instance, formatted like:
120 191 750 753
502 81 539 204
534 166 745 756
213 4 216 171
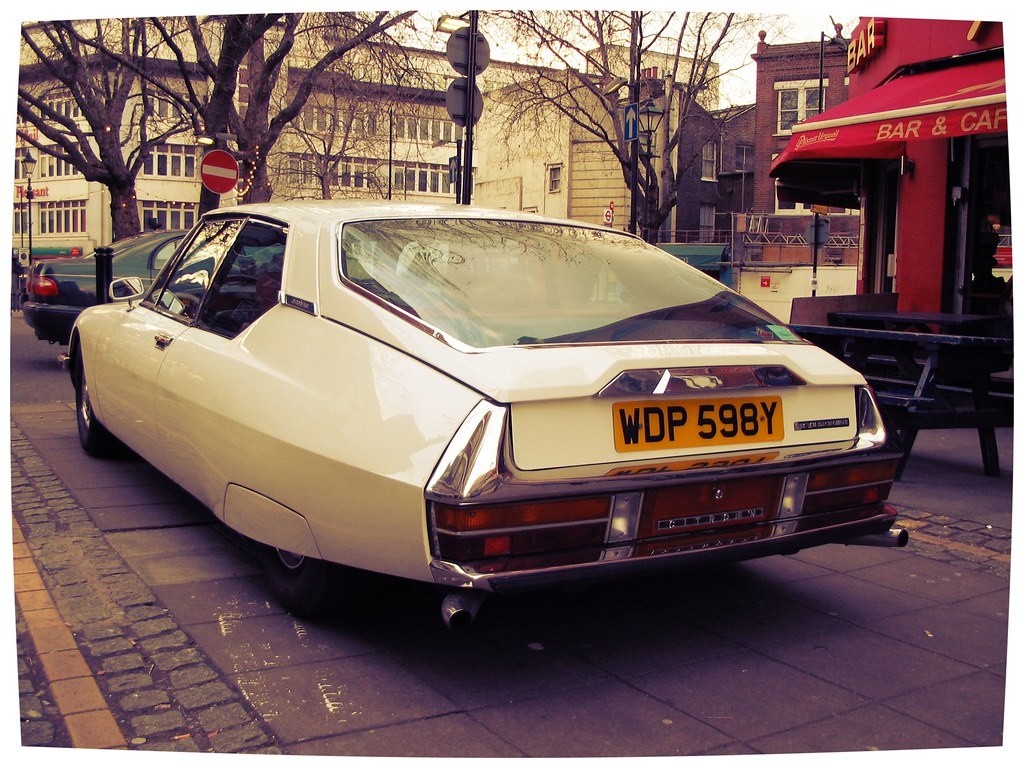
863 375 1013 407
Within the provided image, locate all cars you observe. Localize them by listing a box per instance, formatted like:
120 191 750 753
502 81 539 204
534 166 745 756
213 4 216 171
18 228 247 346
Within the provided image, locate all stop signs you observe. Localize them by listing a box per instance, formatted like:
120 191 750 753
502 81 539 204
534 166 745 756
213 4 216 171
200 150 240 196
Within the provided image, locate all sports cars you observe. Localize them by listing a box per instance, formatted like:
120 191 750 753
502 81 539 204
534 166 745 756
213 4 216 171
65 200 912 631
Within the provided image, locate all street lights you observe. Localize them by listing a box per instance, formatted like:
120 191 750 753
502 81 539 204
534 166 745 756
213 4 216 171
601 78 637 234
357 96 393 201
16 184 24 247
808 31 848 297
21 149 36 270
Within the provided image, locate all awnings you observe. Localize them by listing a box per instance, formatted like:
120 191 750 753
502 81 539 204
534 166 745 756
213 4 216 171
769 56 1009 206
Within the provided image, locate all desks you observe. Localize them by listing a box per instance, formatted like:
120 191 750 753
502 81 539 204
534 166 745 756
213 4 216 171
826 311 997 334
786 324 1014 482
